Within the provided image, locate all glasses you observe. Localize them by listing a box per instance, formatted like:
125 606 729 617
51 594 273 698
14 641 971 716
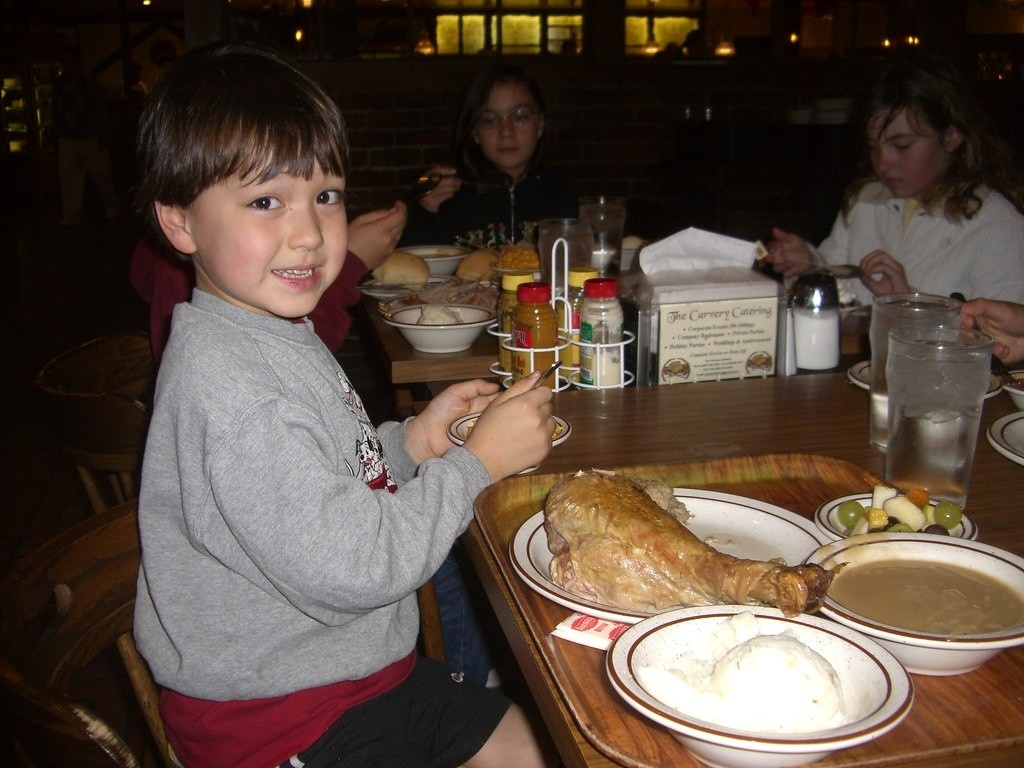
475 108 540 130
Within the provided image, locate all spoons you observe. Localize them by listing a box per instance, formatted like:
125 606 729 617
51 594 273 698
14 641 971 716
403 172 441 205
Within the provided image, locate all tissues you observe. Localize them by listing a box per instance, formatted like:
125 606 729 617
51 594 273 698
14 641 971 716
626 226 779 386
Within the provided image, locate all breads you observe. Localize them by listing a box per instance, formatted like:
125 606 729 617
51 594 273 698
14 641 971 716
456 250 498 280
372 252 428 286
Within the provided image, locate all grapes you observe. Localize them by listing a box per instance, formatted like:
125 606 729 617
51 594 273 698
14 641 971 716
837 500 864 526
935 500 963 529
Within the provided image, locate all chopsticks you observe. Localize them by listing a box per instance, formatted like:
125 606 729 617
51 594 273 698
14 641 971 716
355 282 443 289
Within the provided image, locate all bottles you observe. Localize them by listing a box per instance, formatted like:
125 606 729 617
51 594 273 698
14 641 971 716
579 278 623 387
496 271 535 389
556 267 599 380
509 283 558 389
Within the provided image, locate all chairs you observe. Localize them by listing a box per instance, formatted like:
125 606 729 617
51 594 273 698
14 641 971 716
28 327 152 513
0 499 446 768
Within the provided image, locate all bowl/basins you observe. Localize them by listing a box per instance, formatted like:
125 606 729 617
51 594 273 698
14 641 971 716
489 260 542 282
446 411 573 475
382 303 496 353
1001 370 1024 411
604 602 915 768
395 244 472 277
620 250 639 272
805 533 1024 676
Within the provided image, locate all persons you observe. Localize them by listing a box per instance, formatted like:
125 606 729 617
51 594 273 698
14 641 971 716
756 54 1024 304
395 62 616 249
44 44 119 231
107 39 559 768
126 194 411 428
960 297 1024 370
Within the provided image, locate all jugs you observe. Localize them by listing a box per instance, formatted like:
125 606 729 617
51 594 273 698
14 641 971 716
787 273 839 309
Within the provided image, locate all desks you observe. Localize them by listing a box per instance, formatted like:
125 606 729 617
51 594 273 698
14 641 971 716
410 369 1024 768
356 236 873 664
672 117 868 244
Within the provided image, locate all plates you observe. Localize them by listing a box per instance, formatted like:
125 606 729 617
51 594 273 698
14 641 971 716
838 300 862 312
361 274 456 302
507 487 832 625
986 410 1024 466
814 495 979 543
377 298 401 314
847 360 1003 400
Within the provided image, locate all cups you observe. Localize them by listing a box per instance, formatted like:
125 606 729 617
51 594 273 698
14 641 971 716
537 217 594 296
885 324 993 508
577 196 625 278
868 292 961 452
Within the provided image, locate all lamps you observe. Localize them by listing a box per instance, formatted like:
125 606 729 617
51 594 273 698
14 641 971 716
414 30 434 55
